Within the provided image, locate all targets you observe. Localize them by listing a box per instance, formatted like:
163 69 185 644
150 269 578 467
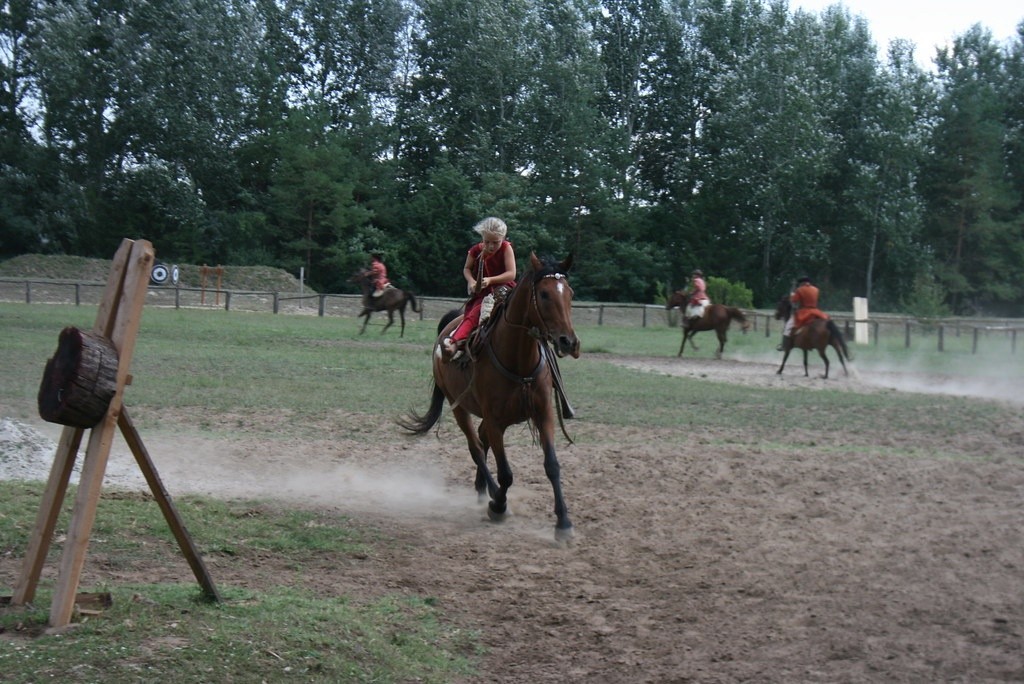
150 265 169 284
170 265 179 285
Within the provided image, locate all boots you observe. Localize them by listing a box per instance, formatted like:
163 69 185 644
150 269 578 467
776 334 792 351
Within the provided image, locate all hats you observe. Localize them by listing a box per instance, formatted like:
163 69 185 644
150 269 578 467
797 276 810 282
691 269 703 275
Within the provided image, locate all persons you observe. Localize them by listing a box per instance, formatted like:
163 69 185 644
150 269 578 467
441 217 516 363
777 275 828 352
676 269 712 329
358 253 393 316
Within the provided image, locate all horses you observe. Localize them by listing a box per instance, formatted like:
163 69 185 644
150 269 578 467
774 293 852 380
394 248 582 549
346 266 423 339
666 288 748 360
